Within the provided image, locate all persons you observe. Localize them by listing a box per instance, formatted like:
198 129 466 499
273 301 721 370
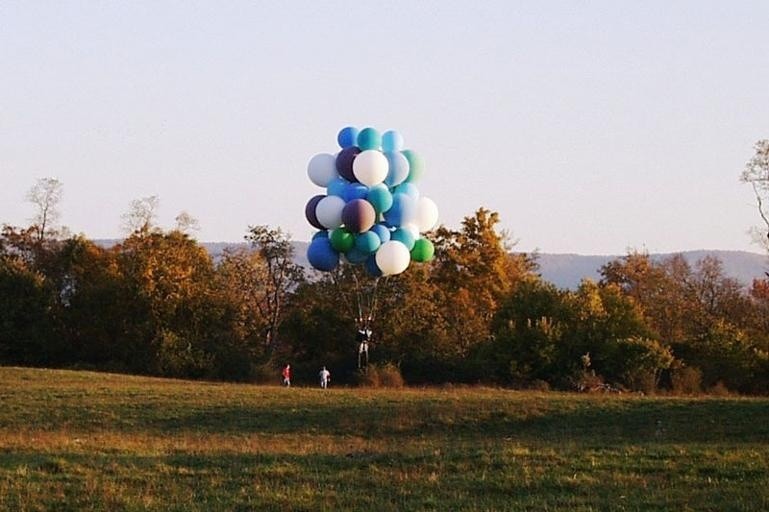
282 364 292 388
319 365 331 390
357 325 373 369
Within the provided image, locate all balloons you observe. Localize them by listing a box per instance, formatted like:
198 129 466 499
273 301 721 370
306 125 439 279
